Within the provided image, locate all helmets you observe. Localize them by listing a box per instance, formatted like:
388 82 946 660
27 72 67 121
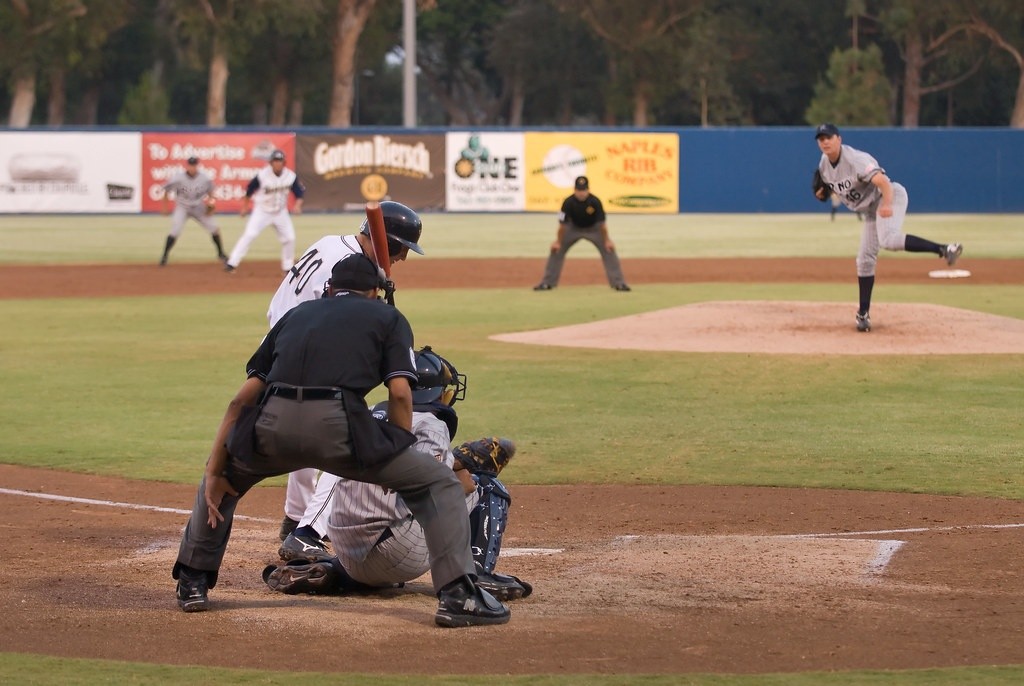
412 350 467 407
331 255 386 290
361 201 425 256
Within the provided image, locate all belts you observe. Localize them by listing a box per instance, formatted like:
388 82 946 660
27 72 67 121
269 386 343 400
373 527 393 546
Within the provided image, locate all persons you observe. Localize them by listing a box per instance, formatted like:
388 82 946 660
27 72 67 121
172 253 510 627
813 125 963 331
533 176 631 291
225 150 308 274
159 156 229 266
266 201 425 562
264 344 533 601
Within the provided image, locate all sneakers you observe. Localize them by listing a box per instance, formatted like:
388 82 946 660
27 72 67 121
278 532 337 561
176 571 211 613
435 583 511 628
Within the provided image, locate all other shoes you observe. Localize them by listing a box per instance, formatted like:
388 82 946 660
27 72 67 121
161 254 167 265
279 514 300 541
945 244 963 266
219 252 228 260
855 311 872 331
534 283 551 290
615 283 631 291
476 574 525 602
223 264 235 272
268 563 333 594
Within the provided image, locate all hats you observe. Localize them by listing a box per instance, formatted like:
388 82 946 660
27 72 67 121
272 151 285 160
813 123 839 139
575 176 588 189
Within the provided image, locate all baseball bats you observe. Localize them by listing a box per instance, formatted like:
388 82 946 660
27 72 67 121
364 201 395 306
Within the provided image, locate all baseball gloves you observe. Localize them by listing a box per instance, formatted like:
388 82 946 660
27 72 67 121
453 435 518 477
208 198 216 213
